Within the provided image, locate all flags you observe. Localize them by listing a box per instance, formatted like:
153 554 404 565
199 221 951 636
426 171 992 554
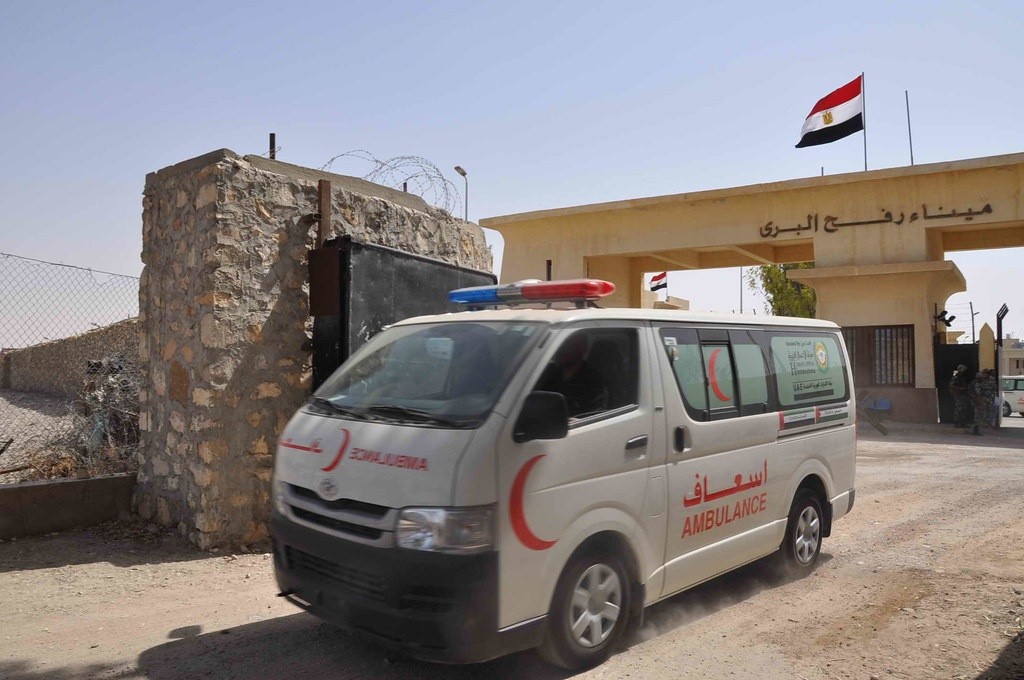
795 76 863 148
650 271 667 291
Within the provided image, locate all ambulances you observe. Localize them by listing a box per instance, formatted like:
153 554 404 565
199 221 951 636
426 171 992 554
1001 375 1024 418
267 279 857 672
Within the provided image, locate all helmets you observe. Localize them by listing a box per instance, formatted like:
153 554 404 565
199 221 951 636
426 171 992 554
957 364 967 372
976 372 986 382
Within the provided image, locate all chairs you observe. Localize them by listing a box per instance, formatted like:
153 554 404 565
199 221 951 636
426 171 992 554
586 340 628 406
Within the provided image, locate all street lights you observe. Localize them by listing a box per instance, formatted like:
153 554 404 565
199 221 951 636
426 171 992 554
970 302 980 344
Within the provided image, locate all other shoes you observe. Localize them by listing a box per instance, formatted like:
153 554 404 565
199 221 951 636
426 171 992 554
955 423 969 428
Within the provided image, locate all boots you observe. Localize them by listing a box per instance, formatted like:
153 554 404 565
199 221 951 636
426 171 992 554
973 425 982 436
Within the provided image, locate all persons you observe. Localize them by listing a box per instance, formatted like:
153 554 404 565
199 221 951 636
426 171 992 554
546 332 609 421
972 368 997 435
950 364 976 429
